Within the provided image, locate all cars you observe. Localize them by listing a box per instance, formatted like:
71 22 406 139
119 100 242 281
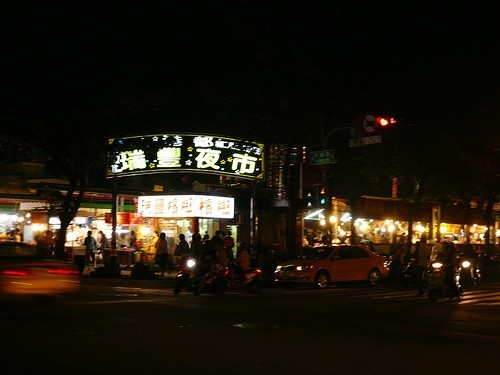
274 245 392 289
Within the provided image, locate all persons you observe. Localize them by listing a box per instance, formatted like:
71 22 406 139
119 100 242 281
33 230 61 255
362 232 497 300
98 231 108 264
111 231 137 249
154 233 172 276
174 234 189 258
304 228 331 246
6 228 19 240
238 243 250 272
84 231 97 271
191 230 234 258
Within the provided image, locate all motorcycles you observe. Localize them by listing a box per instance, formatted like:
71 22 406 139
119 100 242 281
424 242 465 304
458 247 477 293
400 255 417 287
172 251 260 299
